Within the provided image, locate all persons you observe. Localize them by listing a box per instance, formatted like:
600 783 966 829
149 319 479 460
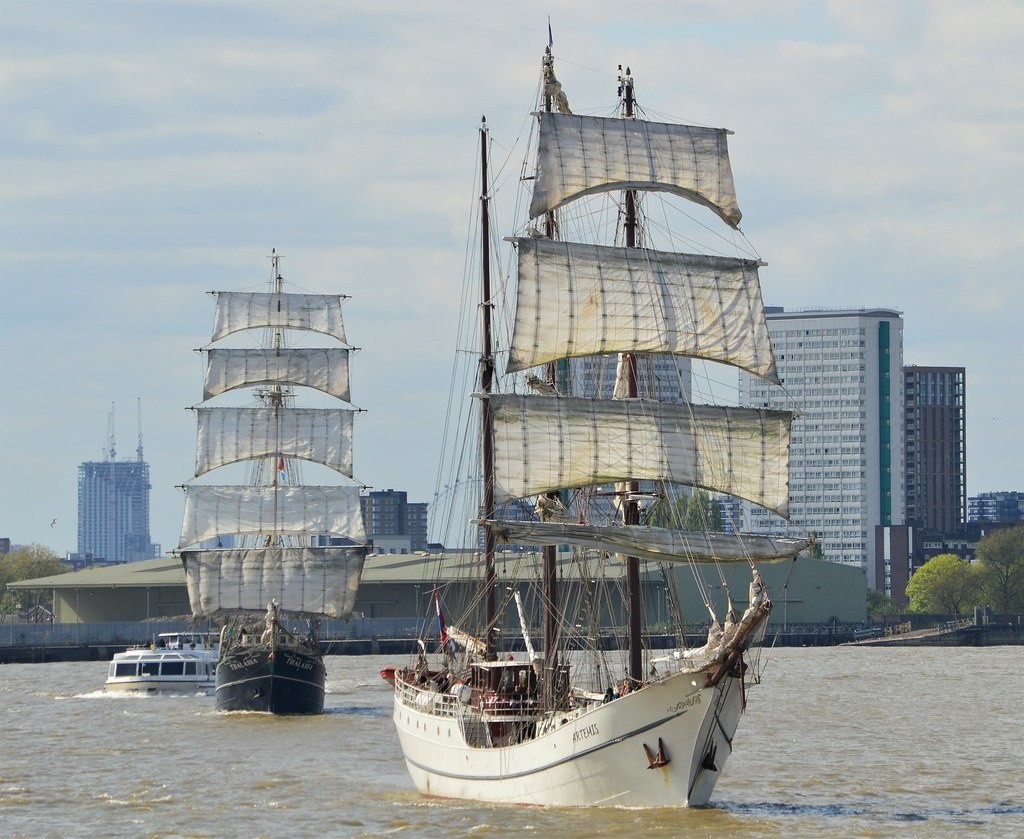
238 624 259 647
142 638 217 650
409 653 633 704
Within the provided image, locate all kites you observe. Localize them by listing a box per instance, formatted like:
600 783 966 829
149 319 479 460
435 590 449 651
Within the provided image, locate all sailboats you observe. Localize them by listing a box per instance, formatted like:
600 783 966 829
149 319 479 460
162 246 385 717
392 12 818 812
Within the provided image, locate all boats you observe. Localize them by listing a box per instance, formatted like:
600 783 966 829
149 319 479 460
377 665 437 691
100 632 218 694
